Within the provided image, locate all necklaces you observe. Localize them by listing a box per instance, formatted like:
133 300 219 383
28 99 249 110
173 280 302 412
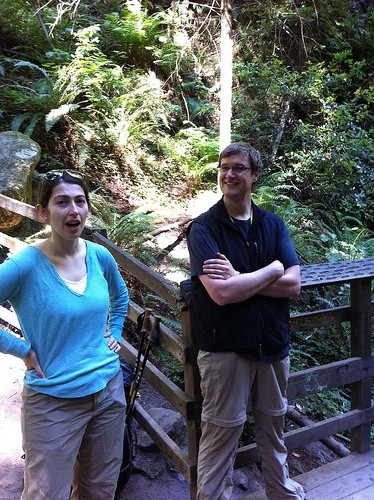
227 206 258 251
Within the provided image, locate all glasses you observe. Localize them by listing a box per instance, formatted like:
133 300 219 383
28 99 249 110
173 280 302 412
216 166 255 173
41 169 87 181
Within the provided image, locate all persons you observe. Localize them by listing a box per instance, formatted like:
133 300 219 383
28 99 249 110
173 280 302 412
187 141 308 500
0 170 129 500
